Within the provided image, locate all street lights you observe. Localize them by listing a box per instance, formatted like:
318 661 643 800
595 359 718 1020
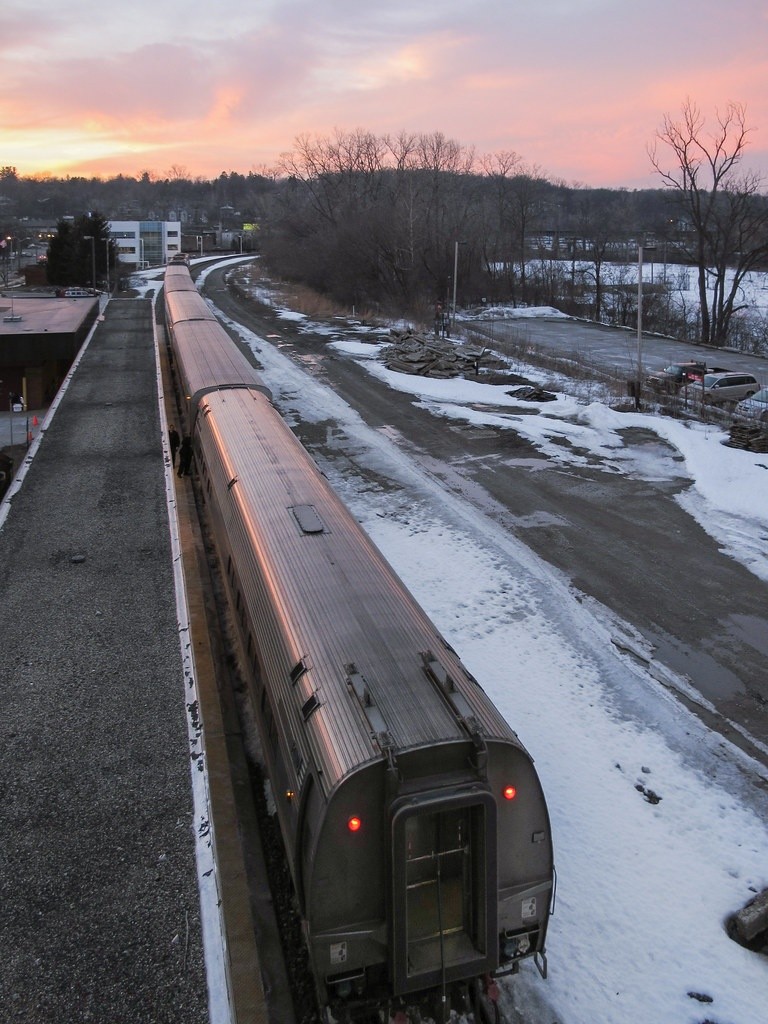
101 237 109 296
83 236 97 296
9 392 23 478
6 236 14 274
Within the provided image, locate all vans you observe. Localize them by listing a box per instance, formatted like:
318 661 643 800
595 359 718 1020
680 372 760 405
735 387 768 421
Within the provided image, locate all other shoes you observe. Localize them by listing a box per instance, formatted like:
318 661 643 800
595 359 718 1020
177 473 182 478
183 473 190 476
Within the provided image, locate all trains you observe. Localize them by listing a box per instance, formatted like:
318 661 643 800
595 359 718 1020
163 253 555 1024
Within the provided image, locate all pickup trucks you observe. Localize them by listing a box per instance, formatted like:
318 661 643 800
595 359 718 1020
645 361 733 396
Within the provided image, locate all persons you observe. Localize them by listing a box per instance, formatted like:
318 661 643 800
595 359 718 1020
169 424 180 469
177 440 194 478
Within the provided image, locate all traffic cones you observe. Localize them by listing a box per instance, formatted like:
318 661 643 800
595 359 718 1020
28 431 33 440
32 416 39 425
